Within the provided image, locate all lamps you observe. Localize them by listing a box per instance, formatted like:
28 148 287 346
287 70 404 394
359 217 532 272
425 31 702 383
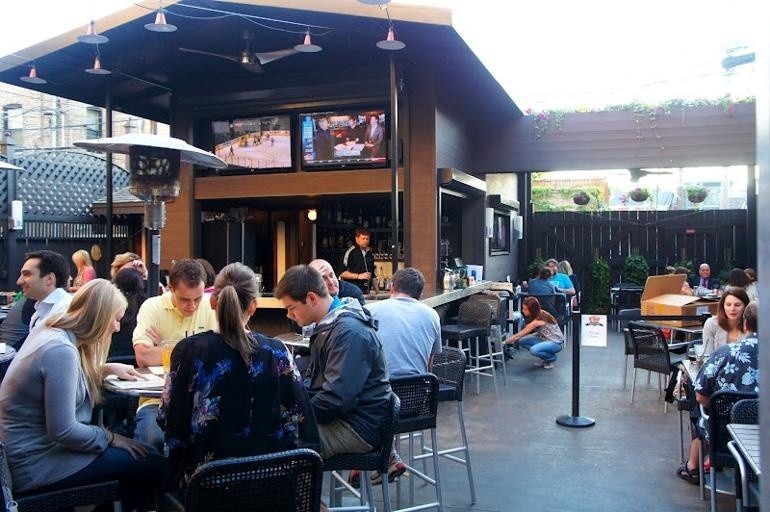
376 7 406 51
77 20 110 45
294 27 323 53
19 63 47 85
85 57 112 75
144 6 178 33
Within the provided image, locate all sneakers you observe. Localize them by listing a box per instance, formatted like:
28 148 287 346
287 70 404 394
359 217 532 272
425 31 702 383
370 453 406 485
544 361 552 369
348 473 360 488
533 358 544 366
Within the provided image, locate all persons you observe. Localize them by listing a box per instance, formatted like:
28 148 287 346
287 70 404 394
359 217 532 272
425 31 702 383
528 268 559 320
112 253 149 282
364 114 384 158
703 289 750 353
228 123 275 158
109 268 148 357
725 269 755 300
274 264 392 460
744 268 757 285
317 118 332 160
0 296 37 352
309 259 365 306
556 260 577 289
17 250 73 333
132 259 220 366
690 263 720 290
506 297 564 369
678 302 759 485
340 228 380 294
68 249 96 293
195 259 215 294
156 263 322 504
0 278 159 499
545 259 575 294
346 116 362 144
665 265 675 275
675 267 693 295
363 268 442 484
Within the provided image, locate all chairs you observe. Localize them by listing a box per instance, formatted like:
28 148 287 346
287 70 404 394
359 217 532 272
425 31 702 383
449 294 507 386
616 287 643 333
0 434 124 512
726 398 760 512
164 448 324 512
390 371 444 512
441 300 497 395
322 390 403 512
618 308 687 392
628 320 684 415
520 293 569 347
395 345 477 507
696 389 758 512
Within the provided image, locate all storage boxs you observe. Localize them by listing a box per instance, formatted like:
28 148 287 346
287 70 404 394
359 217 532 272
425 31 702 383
639 274 720 327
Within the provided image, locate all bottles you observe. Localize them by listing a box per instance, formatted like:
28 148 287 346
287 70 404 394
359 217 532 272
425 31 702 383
379 267 384 287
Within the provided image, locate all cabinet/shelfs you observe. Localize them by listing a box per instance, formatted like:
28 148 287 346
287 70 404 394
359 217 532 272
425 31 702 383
360 279 492 345
319 207 404 290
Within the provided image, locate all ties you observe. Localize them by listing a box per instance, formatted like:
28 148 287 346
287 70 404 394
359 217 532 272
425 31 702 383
702 278 706 288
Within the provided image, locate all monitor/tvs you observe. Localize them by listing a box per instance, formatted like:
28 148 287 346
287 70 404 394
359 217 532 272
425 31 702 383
299 103 392 171
207 110 297 175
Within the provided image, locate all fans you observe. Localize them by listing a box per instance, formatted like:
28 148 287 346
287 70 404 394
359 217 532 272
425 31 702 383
178 21 301 74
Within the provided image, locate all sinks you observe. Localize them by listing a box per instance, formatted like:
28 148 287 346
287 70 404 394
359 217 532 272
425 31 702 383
365 295 390 300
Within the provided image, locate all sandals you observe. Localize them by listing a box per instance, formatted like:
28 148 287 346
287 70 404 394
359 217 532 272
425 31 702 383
703 463 710 473
677 461 705 485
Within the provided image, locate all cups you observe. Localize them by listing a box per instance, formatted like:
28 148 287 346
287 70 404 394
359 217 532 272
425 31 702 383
161 340 178 379
